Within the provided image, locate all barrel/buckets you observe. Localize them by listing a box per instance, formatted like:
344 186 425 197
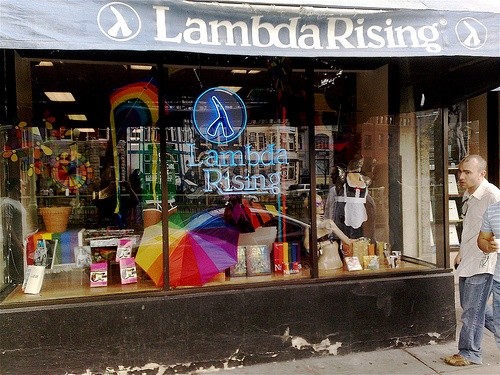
38 207 72 232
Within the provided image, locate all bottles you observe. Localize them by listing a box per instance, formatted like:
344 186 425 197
281 163 287 178
258 132 265 150
281 132 286 148
289 163 294 177
289 133 295 148
249 132 256 150
269 132 276 150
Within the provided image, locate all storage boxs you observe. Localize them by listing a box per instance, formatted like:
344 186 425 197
89 240 138 288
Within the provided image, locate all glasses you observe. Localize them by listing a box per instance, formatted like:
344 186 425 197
315 201 325 207
458 198 469 216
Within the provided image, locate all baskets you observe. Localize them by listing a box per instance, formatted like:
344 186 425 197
143 196 177 229
37 207 72 233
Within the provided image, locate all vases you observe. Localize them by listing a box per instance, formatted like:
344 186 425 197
39 206 72 232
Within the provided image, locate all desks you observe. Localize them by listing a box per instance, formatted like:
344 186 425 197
24 229 85 285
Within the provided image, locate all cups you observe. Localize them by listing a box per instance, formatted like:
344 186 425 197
494 239 500 253
392 251 401 262
389 255 398 268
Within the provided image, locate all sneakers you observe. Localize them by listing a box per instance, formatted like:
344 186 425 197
446 354 472 366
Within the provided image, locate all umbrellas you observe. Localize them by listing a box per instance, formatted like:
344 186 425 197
135 210 241 287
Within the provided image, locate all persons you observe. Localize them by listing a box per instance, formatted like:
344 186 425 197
304 194 351 271
92 144 238 229
333 156 378 242
326 163 424 257
444 155 500 366
0 176 56 287
477 202 500 351
